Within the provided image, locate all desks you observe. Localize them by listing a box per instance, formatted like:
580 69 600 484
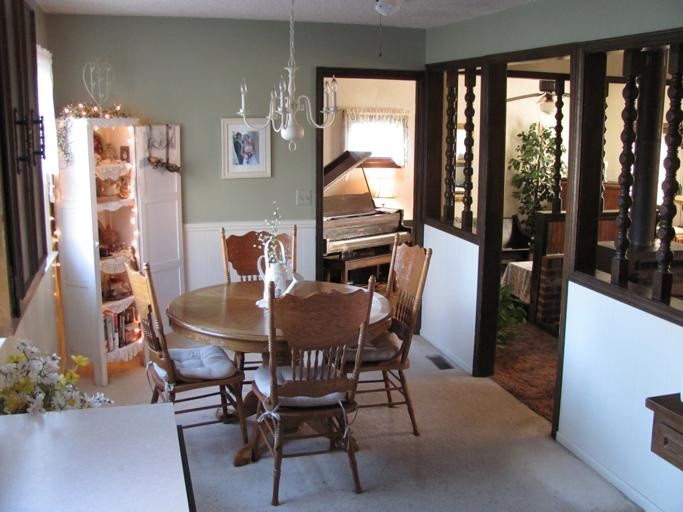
163 277 394 468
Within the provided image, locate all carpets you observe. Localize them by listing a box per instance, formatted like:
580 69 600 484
487 316 558 424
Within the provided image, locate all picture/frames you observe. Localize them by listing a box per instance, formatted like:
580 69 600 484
220 117 272 179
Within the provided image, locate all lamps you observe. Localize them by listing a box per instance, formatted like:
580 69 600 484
539 80 555 114
238 0 339 154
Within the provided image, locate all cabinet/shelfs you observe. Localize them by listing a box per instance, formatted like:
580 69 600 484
54 118 186 386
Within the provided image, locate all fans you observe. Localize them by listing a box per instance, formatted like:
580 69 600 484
506 80 570 114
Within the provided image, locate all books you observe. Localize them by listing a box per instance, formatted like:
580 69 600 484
103 302 139 351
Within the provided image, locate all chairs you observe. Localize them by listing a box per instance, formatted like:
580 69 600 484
214 224 300 399
123 245 248 451
329 231 433 439
249 274 379 505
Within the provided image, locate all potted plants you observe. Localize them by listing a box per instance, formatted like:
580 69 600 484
508 121 568 261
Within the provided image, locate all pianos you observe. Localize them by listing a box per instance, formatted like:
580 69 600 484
324 152 414 285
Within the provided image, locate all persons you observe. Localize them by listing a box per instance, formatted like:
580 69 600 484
239 134 257 165
233 132 243 165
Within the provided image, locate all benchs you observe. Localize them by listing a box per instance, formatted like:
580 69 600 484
453 214 535 265
